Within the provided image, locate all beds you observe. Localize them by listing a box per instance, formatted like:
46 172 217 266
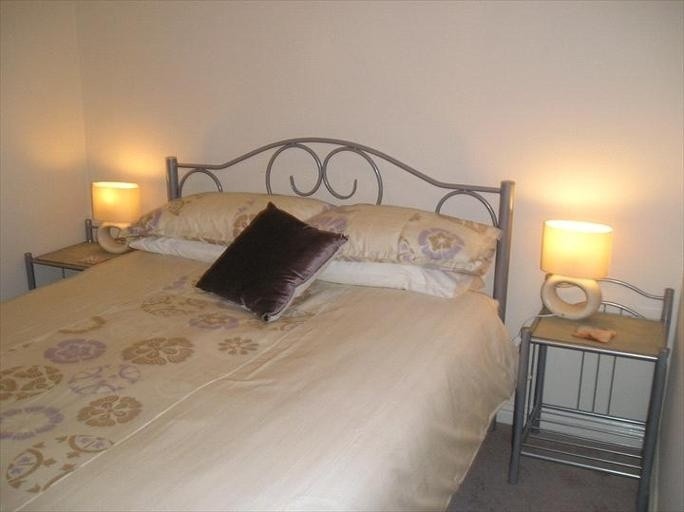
0 140 516 512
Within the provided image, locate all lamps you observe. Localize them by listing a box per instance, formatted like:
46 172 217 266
86 180 142 253
539 219 622 319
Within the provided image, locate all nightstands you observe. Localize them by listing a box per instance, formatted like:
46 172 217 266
23 219 121 288
512 281 677 479
